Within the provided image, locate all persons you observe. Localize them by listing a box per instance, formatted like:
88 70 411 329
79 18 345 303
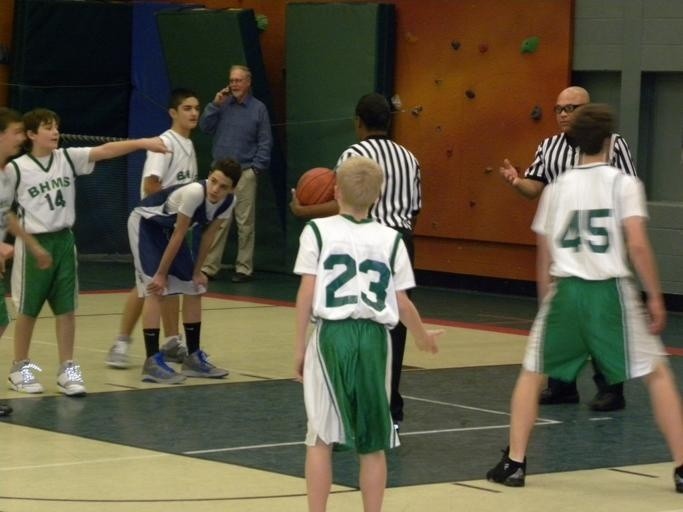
104 91 209 368
500 85 641 411
288 92 424 432
487 102 683 490
291 156 445 511
5 108 175 397
0 108 29 415
127 155 242 384
197 65 273 283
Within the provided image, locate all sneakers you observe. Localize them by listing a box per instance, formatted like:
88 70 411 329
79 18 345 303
7 358 44 394
587 390 626 412
104 340 132 369
181 350 229 378
485 444 528 488
159 334 185 363
54 359 86 396
538 387 580 406
672 463 683 493
140 351 187 384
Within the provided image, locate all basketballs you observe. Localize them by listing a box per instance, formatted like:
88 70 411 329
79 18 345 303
295 167 334 206
